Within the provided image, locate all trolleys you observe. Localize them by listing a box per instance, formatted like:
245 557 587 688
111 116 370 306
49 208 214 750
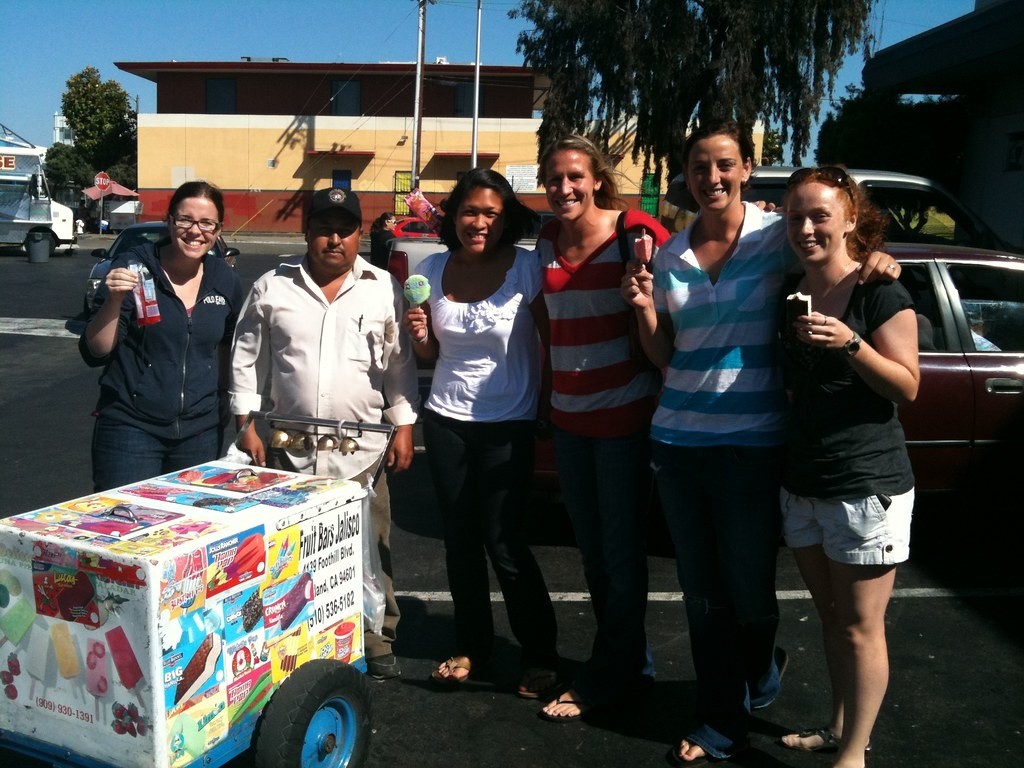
0 410 400 768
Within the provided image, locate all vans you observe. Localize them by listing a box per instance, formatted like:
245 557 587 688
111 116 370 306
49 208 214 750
659 171 1005 248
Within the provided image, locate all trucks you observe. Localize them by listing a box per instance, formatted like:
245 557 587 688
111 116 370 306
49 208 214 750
0 123 78 257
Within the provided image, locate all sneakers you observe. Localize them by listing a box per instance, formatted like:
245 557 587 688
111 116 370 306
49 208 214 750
363 629 401 679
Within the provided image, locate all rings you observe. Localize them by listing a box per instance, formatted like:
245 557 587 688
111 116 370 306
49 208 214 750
887 265 895 271
823 317 826 326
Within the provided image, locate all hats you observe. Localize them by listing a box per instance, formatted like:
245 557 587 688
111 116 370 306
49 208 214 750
305 188 363 229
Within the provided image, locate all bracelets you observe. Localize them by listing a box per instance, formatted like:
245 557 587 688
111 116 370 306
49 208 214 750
413 327 428 343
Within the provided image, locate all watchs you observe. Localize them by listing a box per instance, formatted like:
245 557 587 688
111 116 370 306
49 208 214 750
837 330 860 358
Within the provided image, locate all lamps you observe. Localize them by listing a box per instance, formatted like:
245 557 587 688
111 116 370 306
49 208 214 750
403 136 408 141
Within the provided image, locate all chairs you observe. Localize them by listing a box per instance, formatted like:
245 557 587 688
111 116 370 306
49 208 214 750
915 313 937 353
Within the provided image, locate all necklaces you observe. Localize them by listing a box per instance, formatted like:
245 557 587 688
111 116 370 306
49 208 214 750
833 259 852 288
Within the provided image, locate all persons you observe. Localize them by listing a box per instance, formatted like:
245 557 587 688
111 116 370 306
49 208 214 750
77 200 98 234
79 182 248 491
537 136 784 719
401 169 563 698
369 212 397 270
945 265 1001 352
227 187 422 679
773 164 919 768
621 118 902 768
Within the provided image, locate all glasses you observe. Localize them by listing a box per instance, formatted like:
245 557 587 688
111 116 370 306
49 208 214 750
387 220 397 225
785 167 856 204
165 211 223 235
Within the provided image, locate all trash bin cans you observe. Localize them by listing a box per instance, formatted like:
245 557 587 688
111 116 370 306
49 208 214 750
27 232 50 262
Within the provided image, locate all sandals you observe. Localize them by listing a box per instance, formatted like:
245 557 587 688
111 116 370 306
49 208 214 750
780 726 840 752
431 654 497 685
541 688 596 722
830 736 873 768
608 671 656 692
745 646 789 726
669 737 714 768
516 647 561 698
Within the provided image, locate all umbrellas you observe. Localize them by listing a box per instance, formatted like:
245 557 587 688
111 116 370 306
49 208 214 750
82 180 139 200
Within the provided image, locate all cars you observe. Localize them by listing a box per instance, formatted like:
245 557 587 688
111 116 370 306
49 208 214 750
393 218 439 237
878 242 1024 502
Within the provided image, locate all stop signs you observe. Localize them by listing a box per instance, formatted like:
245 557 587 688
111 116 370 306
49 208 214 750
94 173 110 191
